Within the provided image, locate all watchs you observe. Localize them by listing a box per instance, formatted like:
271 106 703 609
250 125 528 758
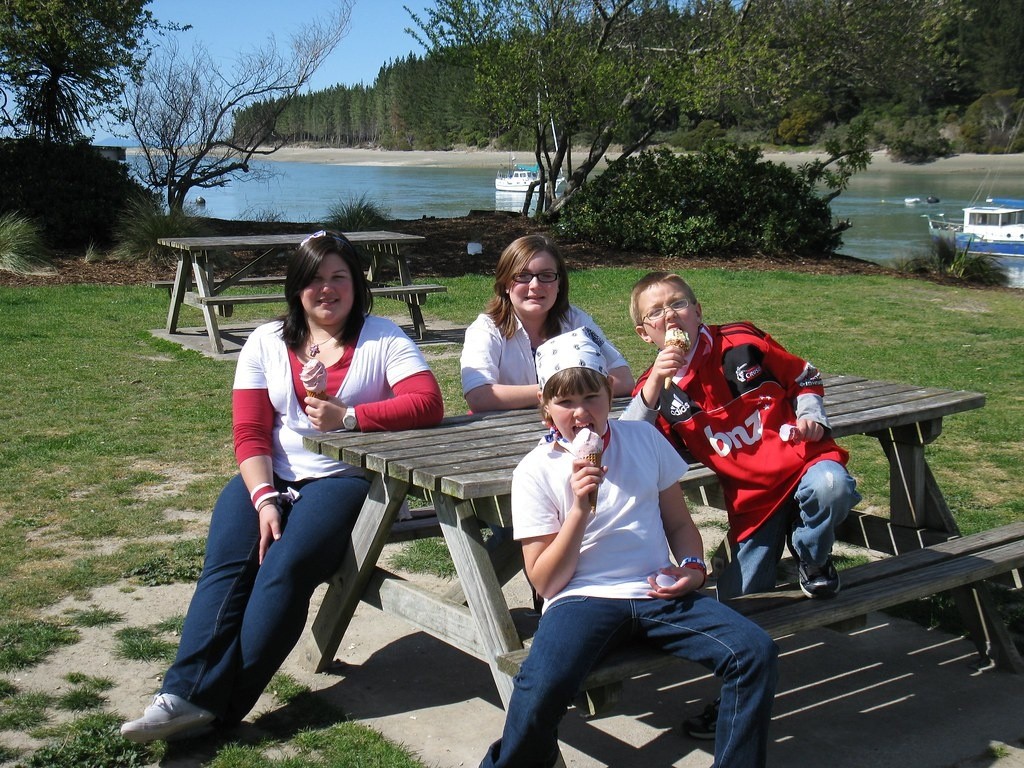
342 405 356 432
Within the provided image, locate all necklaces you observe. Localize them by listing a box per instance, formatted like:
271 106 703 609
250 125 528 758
310 327 344 356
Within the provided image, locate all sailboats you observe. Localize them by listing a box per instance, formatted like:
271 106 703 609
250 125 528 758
494 51 572 193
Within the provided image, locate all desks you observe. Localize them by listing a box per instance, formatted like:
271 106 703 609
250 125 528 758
157 230 426 356
302 375 1024 768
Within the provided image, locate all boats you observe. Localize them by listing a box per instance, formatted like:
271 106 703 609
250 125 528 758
925 97 1024 272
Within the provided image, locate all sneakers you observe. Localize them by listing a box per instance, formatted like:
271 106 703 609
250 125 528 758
121 690 215 742
160 733 213 768
681 695 721 738
788 540 840 599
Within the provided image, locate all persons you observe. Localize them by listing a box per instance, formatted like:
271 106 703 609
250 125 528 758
460 235 635 414
619 272 862 737
121 229 444 741
477 326 780 768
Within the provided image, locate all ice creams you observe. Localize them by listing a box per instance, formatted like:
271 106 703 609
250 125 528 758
572 428 603 517
298 359 327 400
664 327 692 389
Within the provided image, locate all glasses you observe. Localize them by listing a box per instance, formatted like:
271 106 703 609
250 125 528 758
513 270 558 283
641 297 694 326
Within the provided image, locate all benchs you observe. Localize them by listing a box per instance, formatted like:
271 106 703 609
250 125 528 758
150 268 447 317
385 457 1024 695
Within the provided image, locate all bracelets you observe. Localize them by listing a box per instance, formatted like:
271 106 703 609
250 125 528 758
250 484 281 512
680 557 707 590
